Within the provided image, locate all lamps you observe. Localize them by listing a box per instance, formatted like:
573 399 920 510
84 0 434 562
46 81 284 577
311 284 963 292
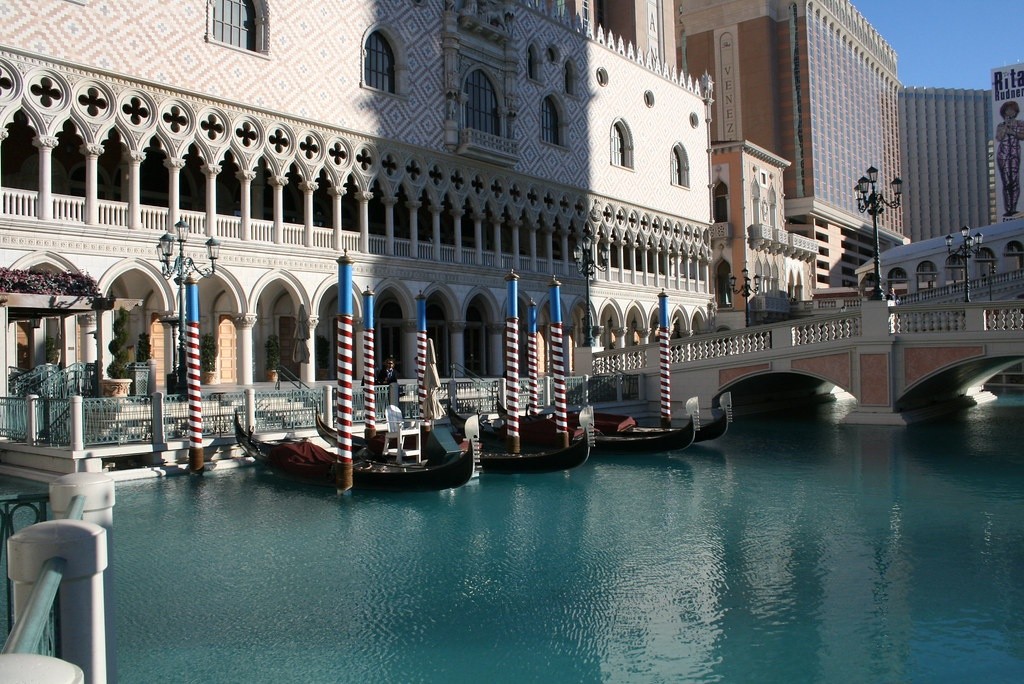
109 287 115 299
607 315 613 328
653 316 659 329
631 313 637 328
582 316 585 326
29 313 42 327
674 316 679 331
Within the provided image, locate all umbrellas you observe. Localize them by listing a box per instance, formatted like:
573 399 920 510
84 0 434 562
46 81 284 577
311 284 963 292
293 305 309 388
423 339 446 430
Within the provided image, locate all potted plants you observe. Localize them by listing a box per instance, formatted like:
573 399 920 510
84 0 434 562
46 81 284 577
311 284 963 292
125 331 152 401
201 332 218 385
46 334 56 399
264 334 281 382
98 306 133 403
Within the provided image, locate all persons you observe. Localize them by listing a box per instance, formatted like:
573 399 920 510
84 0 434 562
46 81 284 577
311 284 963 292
996 101 1024 215
361 359 396 400
885 287 901 318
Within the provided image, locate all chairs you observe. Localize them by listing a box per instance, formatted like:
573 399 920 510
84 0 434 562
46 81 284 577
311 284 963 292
382 404 421 465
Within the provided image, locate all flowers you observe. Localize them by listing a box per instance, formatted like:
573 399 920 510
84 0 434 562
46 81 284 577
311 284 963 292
0 267 100 296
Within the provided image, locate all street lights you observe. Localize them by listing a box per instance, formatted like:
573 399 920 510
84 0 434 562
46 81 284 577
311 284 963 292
730 267 761 328
853 163 904 300
573 231 610 346
155 218 222 404
944 225 984 302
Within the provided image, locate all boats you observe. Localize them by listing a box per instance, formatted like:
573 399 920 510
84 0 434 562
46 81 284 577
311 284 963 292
313 403 598 473
493 388 734 443
445 394 701 454
230 405 476 491
